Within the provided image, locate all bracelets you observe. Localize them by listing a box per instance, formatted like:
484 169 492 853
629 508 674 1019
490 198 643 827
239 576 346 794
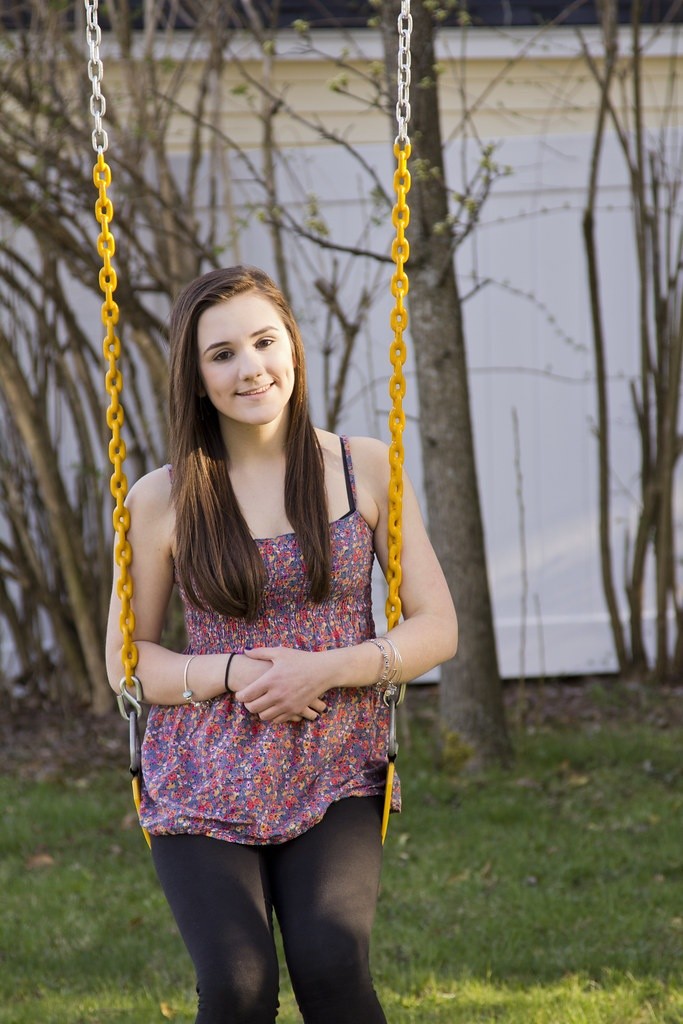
224 652 237 693
366 637 403 697
182 655 216 707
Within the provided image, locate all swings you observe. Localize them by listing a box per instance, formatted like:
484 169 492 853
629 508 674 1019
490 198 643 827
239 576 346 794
80 0 409 852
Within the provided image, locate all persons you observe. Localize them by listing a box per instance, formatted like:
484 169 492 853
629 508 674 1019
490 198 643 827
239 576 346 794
105 265 458 1024
